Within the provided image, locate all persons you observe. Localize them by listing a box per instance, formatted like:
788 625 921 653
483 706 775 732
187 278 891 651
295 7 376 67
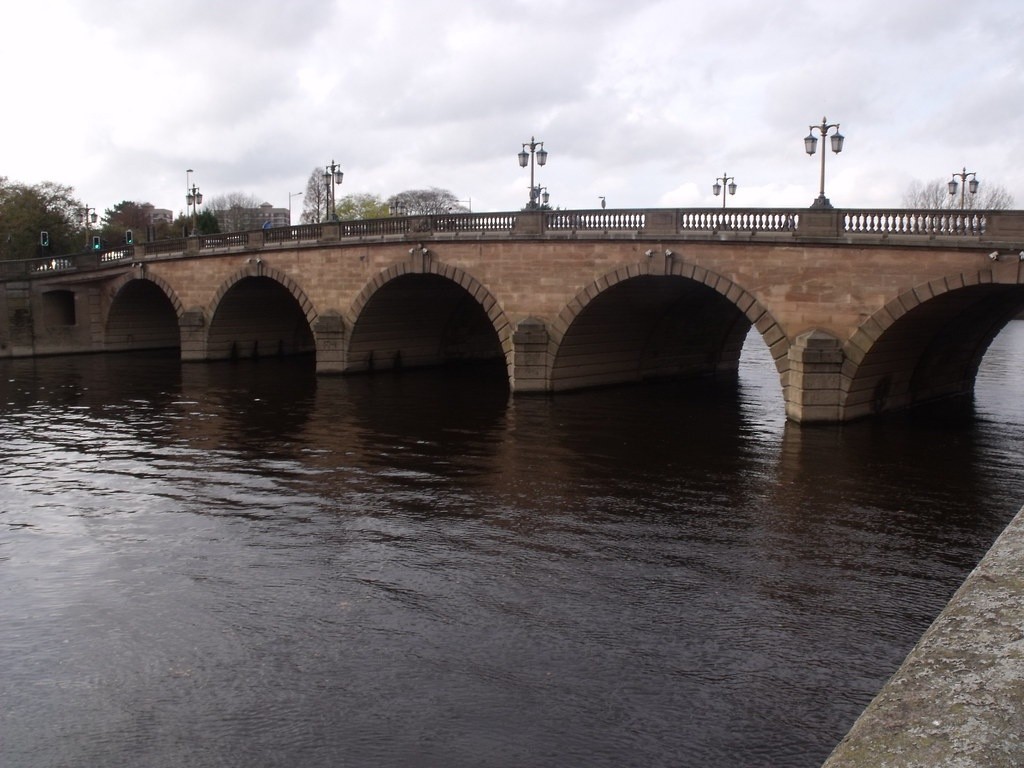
262 217 273 229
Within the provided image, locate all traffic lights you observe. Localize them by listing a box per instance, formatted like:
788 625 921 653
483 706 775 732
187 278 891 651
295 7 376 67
39 230 49 248
92 235 101 250
126 230 132 245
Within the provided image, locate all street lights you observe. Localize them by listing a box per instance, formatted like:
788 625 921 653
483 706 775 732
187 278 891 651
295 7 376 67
288 191 304 223
713 173 736 207
947 168 978 209
75 204 97 250
186 169 201 236
455 198 474 211
517 135 550 208
805 116 843 207
324 158 343 222
